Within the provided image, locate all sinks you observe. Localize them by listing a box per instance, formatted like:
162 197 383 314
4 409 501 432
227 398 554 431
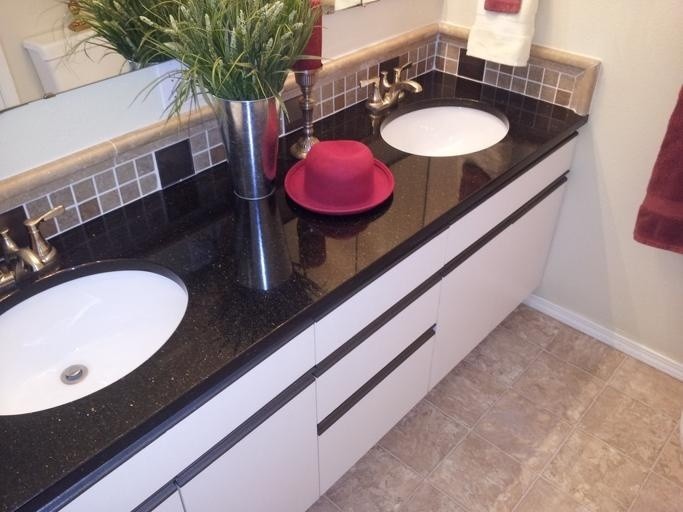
379 97 510 157
0 256 189 417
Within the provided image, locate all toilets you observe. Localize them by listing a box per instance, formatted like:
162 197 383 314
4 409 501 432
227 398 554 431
23 22 131 95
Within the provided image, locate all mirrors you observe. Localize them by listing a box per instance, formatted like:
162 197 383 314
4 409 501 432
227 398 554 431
0 1 208 118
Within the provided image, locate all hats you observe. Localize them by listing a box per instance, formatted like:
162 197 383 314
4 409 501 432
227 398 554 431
283 140 396 216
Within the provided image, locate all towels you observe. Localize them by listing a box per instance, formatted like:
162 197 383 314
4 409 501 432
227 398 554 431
634 82 683 257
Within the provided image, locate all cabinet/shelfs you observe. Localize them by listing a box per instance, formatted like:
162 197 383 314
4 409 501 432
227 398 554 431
53 320 318 512
431 128 578 422
317 227 445 500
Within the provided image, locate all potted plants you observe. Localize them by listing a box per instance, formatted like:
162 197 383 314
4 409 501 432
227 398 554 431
46 2 189 76
129 1 332 201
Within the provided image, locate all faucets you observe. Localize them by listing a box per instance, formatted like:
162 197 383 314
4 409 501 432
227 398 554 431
380 71 423 99
0 226 45 280
